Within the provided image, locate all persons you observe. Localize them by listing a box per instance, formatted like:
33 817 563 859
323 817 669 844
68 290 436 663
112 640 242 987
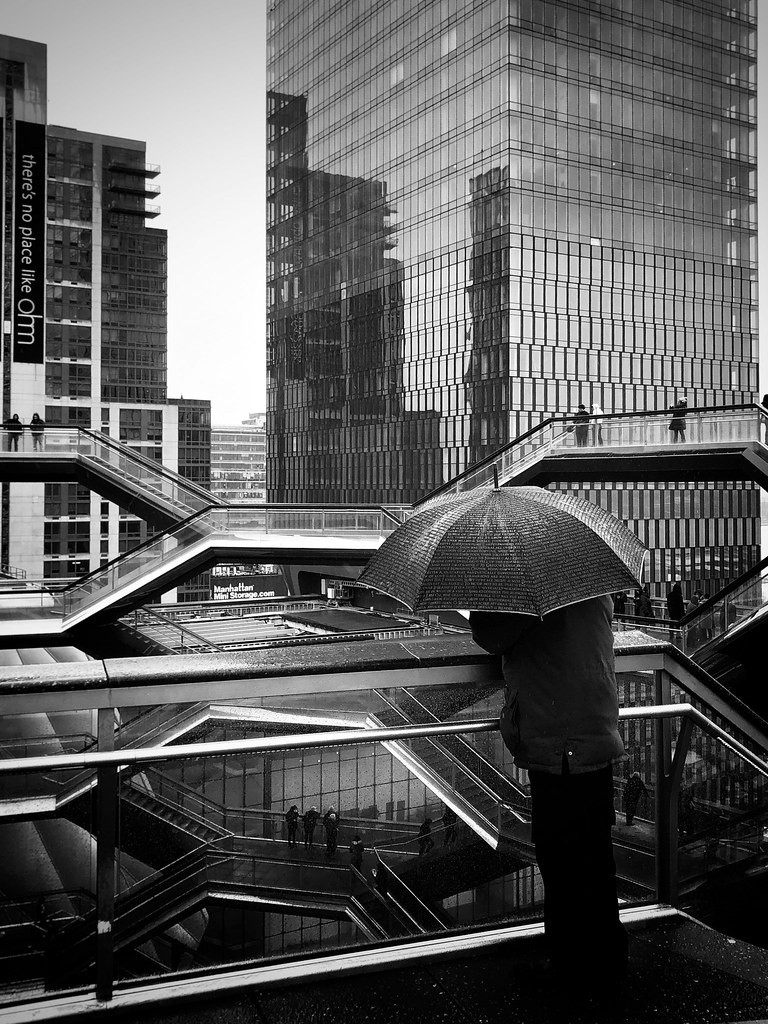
2 413 22 451
417 818 434 856
566 404 590 447
669 397 688 443
589 404 603 447
322 809 339 856
761 393 768 445
470 593 629 1013
30 413 46 452
349 835 364 881
623 771 652 825
285 805 299 848
302 810 317 849
442 806 457 847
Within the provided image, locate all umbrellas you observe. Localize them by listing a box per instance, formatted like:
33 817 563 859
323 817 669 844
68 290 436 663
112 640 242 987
357 463 652 621
